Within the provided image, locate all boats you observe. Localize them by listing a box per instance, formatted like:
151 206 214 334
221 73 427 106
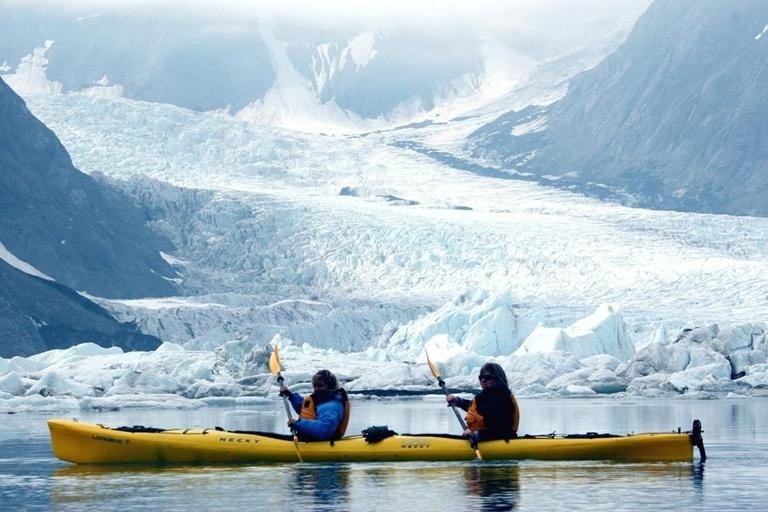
44 414 713 466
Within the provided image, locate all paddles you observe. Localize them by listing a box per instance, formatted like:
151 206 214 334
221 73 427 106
425 350 486 465
269 344 303 464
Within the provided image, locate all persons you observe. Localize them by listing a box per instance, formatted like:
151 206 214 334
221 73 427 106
277 369 351 441
444 362 520 439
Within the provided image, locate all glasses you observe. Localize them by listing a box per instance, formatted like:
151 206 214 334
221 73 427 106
478 374 492 380
313 384 325 388
